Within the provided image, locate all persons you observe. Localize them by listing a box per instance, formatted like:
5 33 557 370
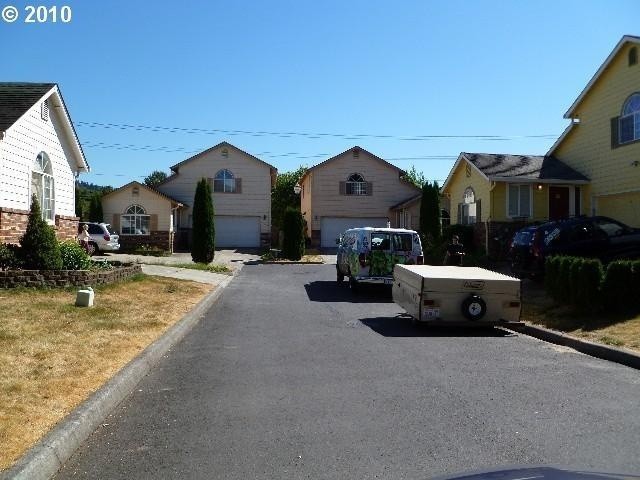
81 224 93 254
446 235 465 266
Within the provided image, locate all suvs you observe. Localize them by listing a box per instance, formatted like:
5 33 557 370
506 213 639 280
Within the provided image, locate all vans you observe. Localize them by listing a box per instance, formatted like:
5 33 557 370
336 226 425 290
79 221 122 255
